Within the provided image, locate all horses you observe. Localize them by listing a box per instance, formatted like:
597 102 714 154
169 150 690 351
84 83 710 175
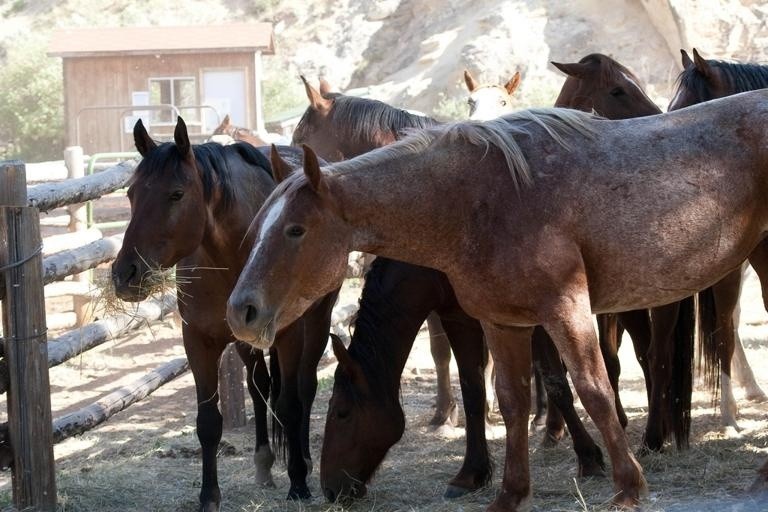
109 47 767 512
